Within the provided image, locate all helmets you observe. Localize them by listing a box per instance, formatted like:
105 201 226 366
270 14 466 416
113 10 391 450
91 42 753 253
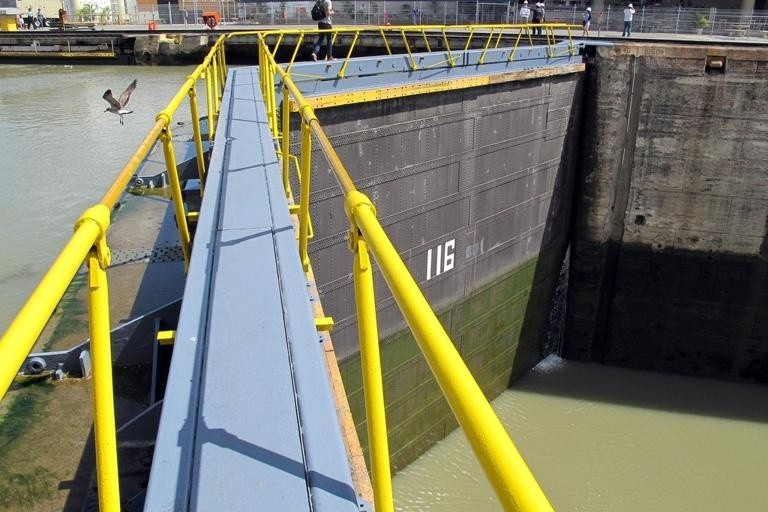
628 3 633 8
535 2 544 8
524 0 528 4
586 6 592 12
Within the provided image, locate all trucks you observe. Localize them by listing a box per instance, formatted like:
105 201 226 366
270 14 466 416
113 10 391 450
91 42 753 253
1 0 63 26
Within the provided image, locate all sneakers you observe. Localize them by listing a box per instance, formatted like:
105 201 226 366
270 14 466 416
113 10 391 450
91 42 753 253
326 55 338 62
311 52 318 62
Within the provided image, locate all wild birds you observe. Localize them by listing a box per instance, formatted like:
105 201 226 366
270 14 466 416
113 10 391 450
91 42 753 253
103 78 138 125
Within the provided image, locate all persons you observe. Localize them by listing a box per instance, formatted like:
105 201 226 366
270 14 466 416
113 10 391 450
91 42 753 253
15 14 27 30
540 3 546 21
621 4 636 38
311 0 338 63
27 8 38 30
519 1 531 36
581 6 592 37
522 2 543 36
37 9 46 30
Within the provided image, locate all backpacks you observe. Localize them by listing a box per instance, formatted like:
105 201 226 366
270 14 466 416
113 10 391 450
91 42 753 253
311 0 326 20
